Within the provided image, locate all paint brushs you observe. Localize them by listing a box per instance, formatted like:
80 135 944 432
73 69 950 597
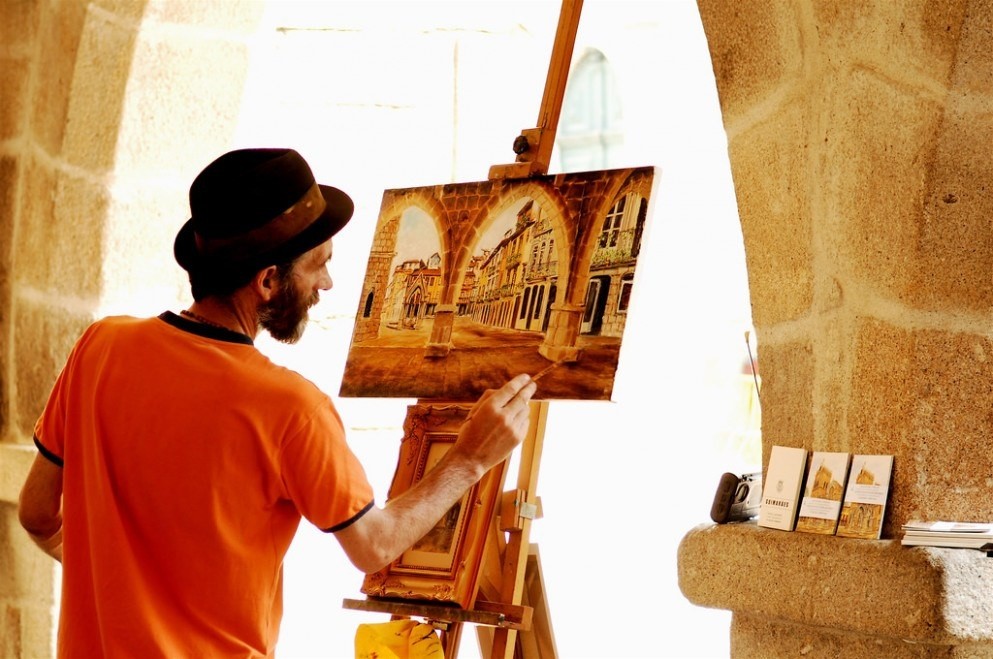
522 343 590 392
744 332 760 395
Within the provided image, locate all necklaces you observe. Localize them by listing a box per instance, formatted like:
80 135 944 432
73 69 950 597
181 309 223 328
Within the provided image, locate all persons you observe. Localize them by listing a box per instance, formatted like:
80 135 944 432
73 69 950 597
17 146 539 659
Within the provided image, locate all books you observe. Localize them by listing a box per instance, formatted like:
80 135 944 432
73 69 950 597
796 449 853 535
836 454 895 540
900 518 993 550
757 445 809 530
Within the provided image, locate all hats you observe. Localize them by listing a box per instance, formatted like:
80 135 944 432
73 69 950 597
174 148 355 271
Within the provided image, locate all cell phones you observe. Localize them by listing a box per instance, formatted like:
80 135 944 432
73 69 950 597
710 464 762 525
